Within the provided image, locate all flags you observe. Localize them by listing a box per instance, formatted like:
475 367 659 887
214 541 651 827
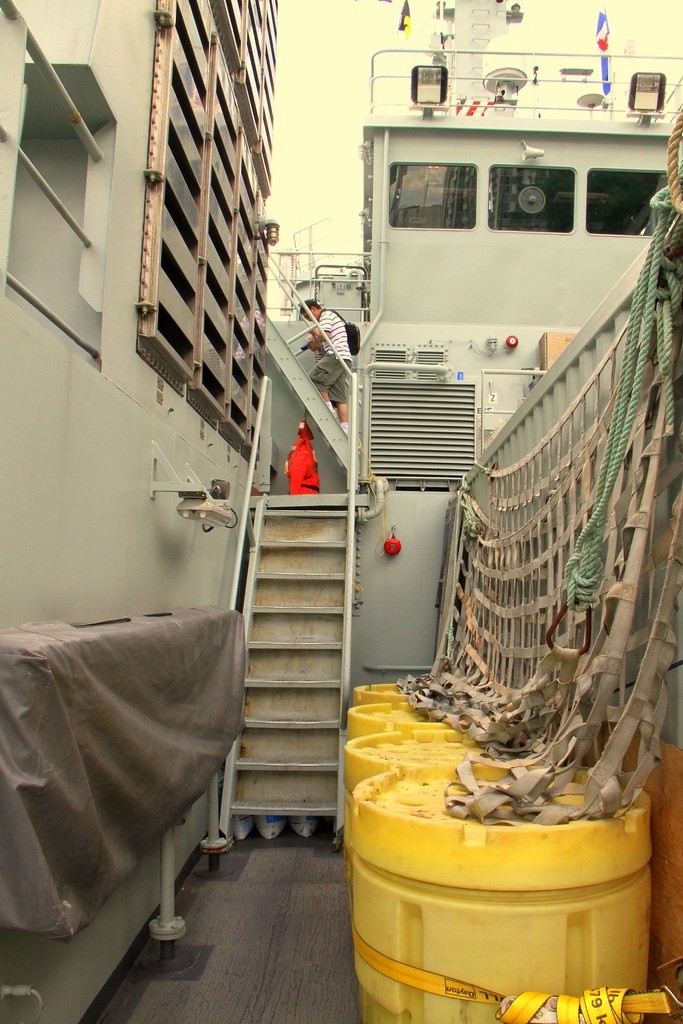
399 0 412 39
596 9 611 95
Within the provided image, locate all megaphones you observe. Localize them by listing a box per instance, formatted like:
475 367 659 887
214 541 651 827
520 140 545 161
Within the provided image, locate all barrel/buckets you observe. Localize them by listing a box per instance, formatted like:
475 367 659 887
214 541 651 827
346 702 467 741
348 763 652 1024
343 728 541 902
352 684 411 708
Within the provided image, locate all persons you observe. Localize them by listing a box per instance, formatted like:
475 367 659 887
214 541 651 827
300 300 353 436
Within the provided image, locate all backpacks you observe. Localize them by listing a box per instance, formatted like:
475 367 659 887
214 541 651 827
318 309 361 356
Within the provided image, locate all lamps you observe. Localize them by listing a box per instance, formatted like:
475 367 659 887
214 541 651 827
411 65 449 111
628 72 666 119
